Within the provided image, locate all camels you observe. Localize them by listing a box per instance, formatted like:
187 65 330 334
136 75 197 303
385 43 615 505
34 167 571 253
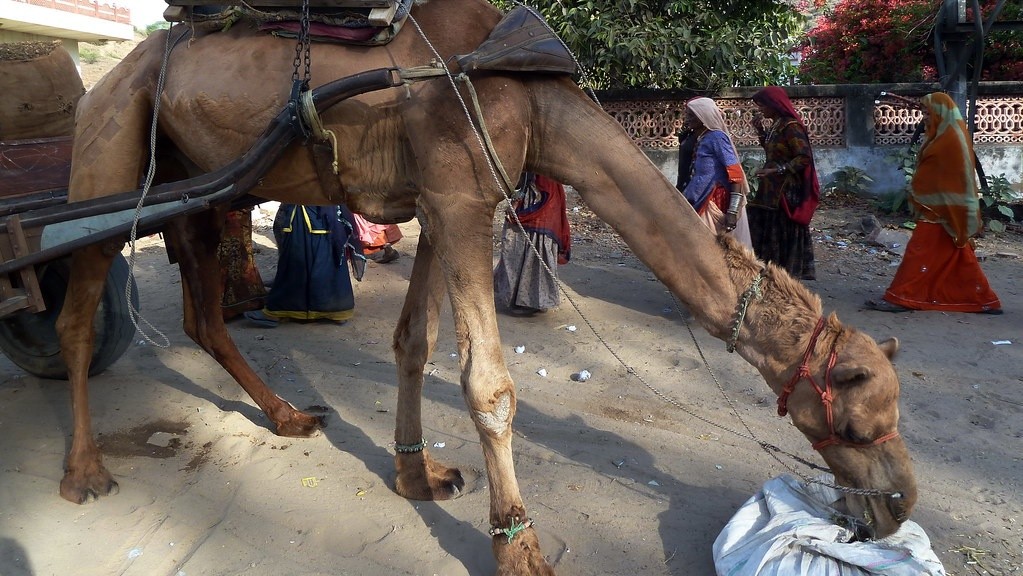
0 0 917 575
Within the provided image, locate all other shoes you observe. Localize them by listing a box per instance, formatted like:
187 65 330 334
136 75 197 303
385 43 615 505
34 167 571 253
511 307 535 314
376 249 399 263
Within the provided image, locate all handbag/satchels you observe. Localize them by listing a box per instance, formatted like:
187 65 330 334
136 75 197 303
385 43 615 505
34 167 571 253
746 203 777 246
700 201 728 236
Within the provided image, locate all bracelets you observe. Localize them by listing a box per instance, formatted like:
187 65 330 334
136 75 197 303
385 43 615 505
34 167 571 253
727 191 744 214
777 165 786 175
680 128 687 134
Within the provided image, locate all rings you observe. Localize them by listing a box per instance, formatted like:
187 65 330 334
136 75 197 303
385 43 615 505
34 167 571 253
727 225 736 230
684 125 686 128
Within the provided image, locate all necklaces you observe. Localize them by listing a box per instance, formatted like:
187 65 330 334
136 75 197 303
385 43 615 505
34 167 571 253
765 118 781 144
697 128 708 141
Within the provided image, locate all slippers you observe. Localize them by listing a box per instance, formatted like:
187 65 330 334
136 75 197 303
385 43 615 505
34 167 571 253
244 310 279 326
866 299 905 310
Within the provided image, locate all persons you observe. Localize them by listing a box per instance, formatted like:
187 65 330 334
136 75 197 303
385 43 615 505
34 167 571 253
677 84 820 322
864 92 1002 315
493 171 571 315
218 199 402 328
